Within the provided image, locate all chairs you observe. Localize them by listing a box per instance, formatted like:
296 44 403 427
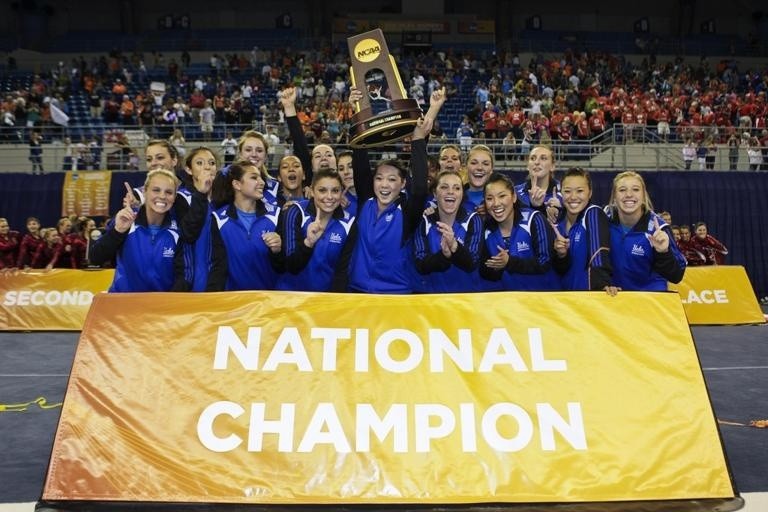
1 24 768 159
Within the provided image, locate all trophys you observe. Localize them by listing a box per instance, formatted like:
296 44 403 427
340 22 424 150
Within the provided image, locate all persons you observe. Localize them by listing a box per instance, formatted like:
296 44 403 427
1 37 767 294
